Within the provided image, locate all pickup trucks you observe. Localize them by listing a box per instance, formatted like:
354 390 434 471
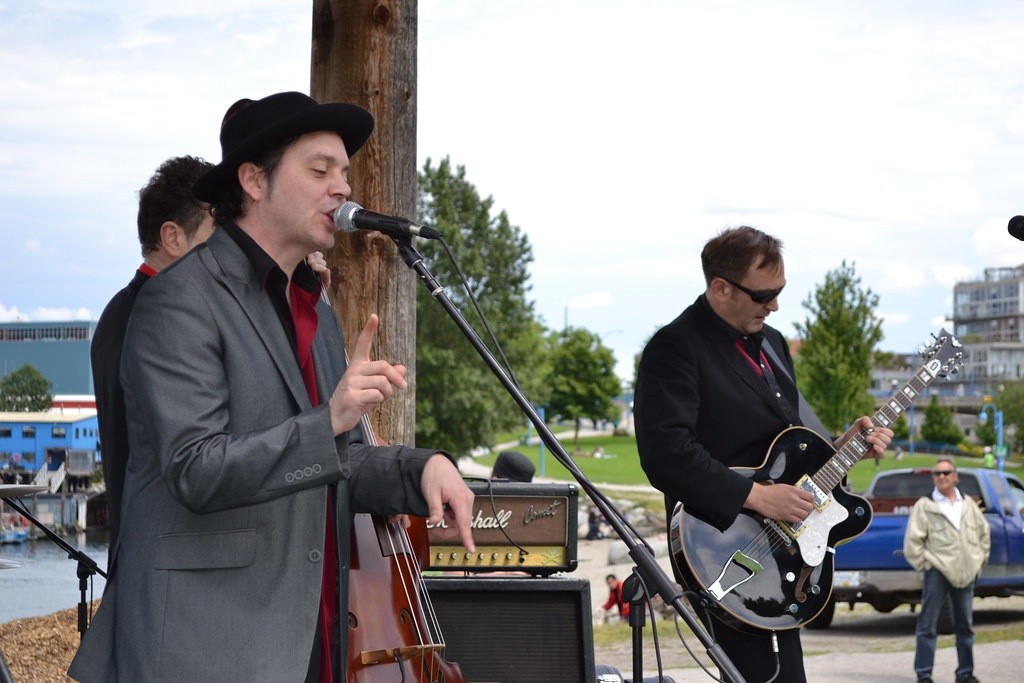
803 466 1024 630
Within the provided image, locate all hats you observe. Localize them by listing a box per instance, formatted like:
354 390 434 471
191 91 375 203
492 451 536 484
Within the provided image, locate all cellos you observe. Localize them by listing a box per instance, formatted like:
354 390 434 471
312 279 468 683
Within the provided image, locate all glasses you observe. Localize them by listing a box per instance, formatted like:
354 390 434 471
724 279 785 305
933 471 952 477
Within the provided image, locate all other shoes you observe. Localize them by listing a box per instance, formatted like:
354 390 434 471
917 677 932 683
955 675 980 683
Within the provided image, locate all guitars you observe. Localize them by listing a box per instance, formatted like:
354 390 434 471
665 326 972 639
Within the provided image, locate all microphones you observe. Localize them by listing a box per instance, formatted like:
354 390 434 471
1007 215 1024 241
333 201 442 239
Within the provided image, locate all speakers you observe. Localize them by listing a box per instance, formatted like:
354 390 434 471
419 576 597 683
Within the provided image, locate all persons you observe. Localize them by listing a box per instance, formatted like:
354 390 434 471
598 573 630 624
88 152 219 578
583 505 602 541
62 88 479 681
902 456 992 683
983 445 995 469
630 224 896 682
601 417 607 432
